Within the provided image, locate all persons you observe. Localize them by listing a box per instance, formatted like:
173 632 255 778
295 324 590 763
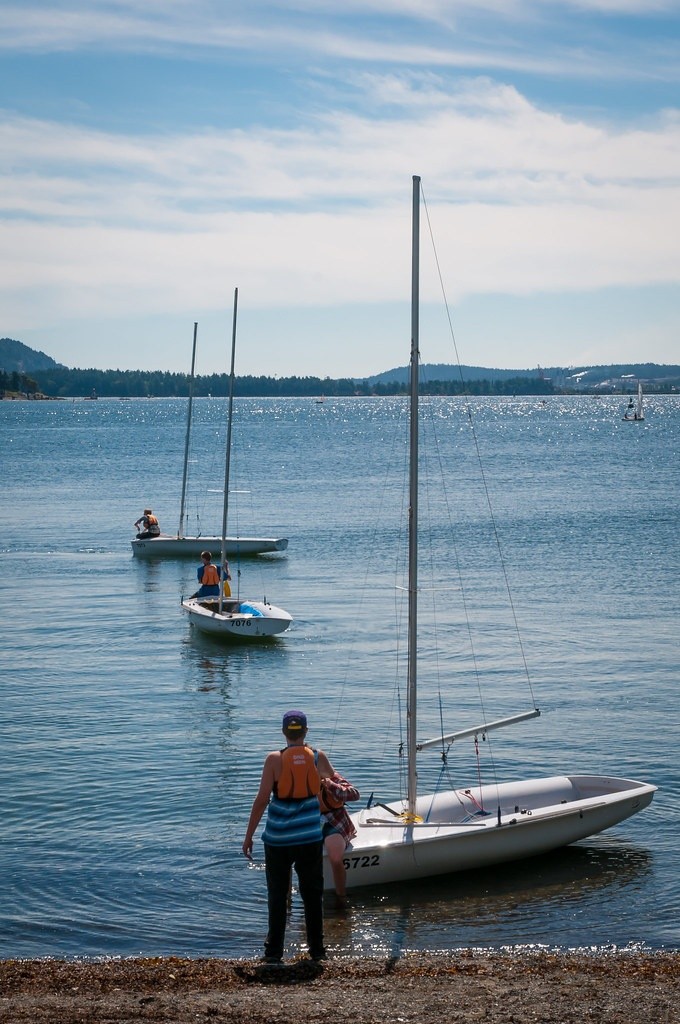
188 551 231 599
321 771 360 897
134 509 161 540
242 711 335 961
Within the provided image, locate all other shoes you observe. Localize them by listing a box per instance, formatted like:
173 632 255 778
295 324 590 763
265 953 280 962
311 953 327 960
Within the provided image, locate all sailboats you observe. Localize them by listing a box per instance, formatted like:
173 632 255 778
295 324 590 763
621 378 646 421
317 175 660 891
130 321 289 558
182 285 293 639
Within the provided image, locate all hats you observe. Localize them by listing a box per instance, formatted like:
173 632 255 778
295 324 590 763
282 711 306 735
